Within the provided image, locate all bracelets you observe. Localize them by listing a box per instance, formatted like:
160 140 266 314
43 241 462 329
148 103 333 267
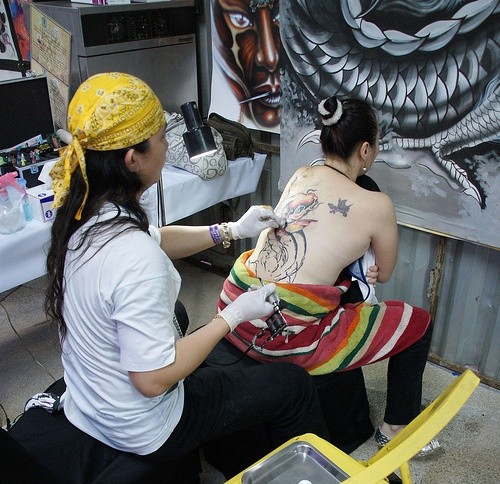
209 223 231 249
213 315 223 321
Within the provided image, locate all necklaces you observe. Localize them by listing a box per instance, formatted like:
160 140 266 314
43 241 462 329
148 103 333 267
323 163 352 180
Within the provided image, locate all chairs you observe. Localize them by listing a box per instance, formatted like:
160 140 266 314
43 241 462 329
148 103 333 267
0 373 206 484
221 369 482 484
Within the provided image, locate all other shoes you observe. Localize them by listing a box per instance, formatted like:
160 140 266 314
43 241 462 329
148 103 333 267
375 427 444 459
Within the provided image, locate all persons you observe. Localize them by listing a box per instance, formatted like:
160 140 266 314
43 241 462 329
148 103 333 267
44 72 331 462
215 94 446 457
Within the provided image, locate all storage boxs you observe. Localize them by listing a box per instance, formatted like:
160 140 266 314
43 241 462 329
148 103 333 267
13 150 60 188
25 184 61 222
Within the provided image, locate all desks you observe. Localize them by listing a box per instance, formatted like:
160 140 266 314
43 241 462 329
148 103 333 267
0 151 268 294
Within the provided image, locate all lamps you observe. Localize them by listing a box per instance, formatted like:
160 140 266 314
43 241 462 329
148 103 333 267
158 101 218 226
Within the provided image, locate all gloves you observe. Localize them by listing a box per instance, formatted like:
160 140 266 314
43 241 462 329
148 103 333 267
216 283 279 335
229 204 285 241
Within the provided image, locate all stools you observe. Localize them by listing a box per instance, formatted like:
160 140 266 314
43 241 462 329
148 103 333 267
202 337 380 479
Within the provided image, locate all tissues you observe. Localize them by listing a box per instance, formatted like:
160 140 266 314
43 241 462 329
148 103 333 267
23 158 60 224
